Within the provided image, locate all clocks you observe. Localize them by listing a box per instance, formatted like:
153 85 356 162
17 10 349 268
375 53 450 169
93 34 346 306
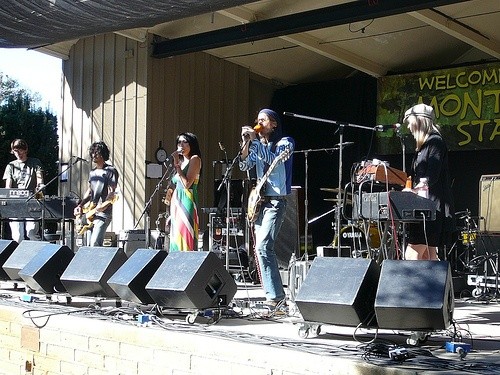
156 147 167 164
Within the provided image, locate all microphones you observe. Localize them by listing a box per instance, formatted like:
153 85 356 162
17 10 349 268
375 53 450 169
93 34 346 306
74 156 90 164
376 123 401 129
243 125 262 137
165 149 182 161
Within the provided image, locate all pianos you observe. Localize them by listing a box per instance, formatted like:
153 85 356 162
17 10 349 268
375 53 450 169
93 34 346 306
351 165 436 262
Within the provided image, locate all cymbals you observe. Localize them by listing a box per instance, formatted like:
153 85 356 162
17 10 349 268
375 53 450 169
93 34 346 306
324 199 351 202
320 188 352 196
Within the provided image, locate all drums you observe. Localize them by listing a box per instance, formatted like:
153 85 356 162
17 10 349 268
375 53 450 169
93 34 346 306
334 224 383 263
461 231 476 245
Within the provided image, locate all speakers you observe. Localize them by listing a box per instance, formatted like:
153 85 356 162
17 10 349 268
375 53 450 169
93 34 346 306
108 247 169 305
148 251 238 311
60 246 127 298
375 259 454 329
0 240 18 280
18 244 74 292
296 257 385 327
1 241 52 282
274 186 313 268
474 235 499 287
479 174 499 234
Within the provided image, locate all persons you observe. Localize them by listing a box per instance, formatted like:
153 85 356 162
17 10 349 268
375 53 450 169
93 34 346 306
2 139 45 244
74 141 119 247
239 109 295 318
403 104 447 260
164 134 202 252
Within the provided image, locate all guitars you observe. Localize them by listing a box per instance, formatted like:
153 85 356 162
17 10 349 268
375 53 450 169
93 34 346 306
76 195 119 236
248 148 291 222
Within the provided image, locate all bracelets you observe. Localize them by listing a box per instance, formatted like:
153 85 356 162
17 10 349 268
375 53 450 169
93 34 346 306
175 161 181 168
179 170 183 176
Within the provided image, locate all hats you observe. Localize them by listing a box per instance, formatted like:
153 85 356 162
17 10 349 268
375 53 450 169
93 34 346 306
402 103 435 123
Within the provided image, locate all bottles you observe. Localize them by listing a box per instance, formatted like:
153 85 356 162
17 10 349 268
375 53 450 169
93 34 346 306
417 178 429 199
290 253 296 262
